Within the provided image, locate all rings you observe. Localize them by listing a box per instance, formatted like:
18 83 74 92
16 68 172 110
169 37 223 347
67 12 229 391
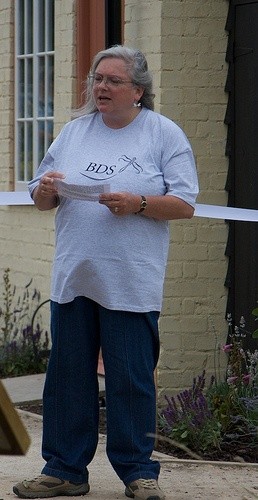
115 207 118 212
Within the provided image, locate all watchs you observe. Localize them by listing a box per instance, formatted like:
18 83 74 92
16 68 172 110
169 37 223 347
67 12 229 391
135 196 147 215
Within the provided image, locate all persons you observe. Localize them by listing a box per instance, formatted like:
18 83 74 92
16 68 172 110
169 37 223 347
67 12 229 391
12 44 199 500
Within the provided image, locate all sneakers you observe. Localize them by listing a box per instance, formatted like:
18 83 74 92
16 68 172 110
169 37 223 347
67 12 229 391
13 474 90 498
124 478 164 500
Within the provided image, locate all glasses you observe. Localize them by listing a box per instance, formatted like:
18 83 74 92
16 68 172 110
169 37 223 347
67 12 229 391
88 74 139 88
